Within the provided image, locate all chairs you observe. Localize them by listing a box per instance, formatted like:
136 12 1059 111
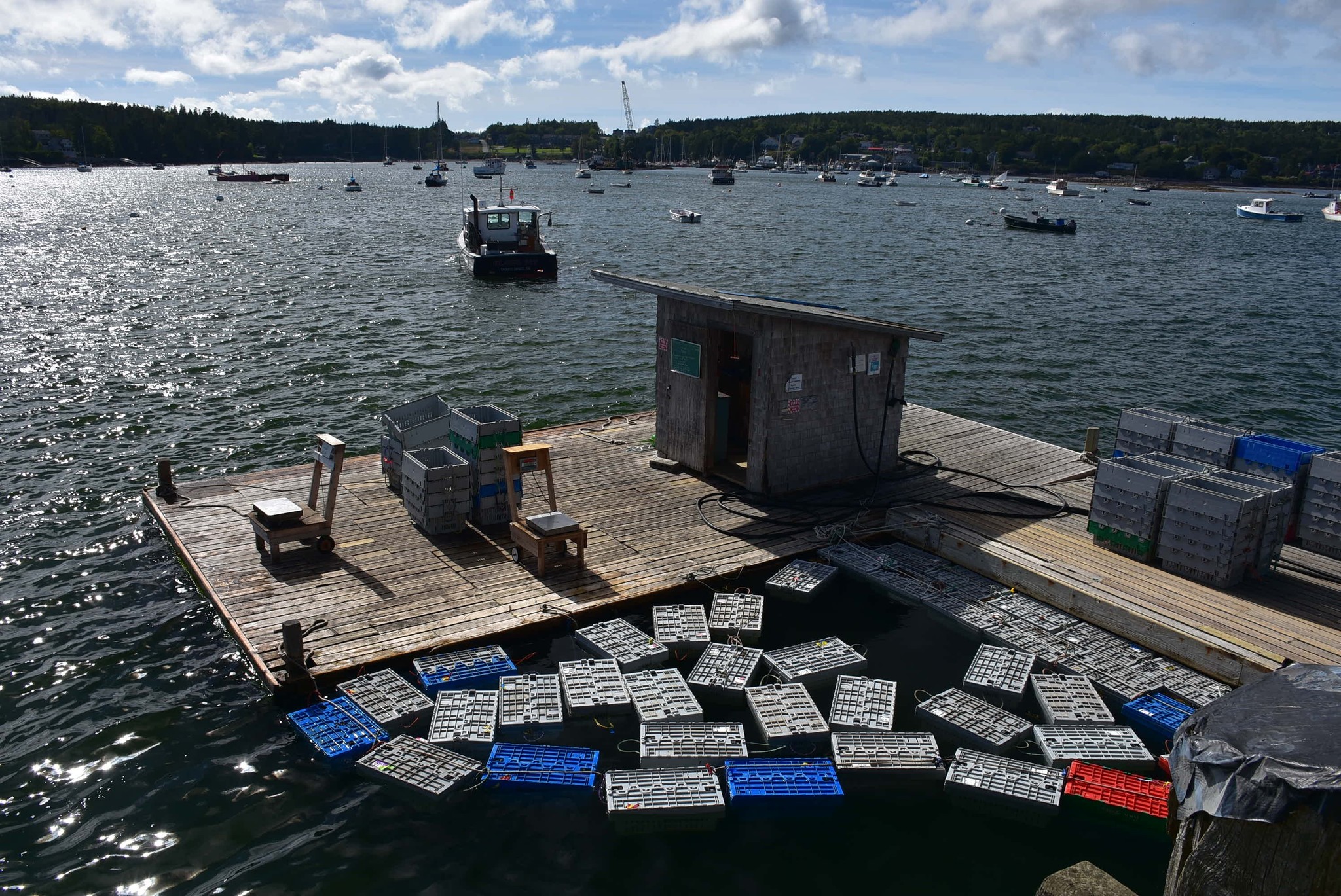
247 433 345 564
502 443 589 577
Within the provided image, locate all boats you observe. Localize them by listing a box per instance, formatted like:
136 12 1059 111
1321 179 1341 221
707 164 735 185
1045 178 1080 196
894 199 919 206
858 168 887 181
1127 198 1152 206
1236 198 1305 222
1133 186 1150 192
424 158 448 187
1079 194 1095 199
622 169 633 174
474 144 508 175
0 165 12 172
857 179 883 187
668 209 703 224
456 152 560 282
818 170 837 182
77 164 93 172
1002 205 1078 236
1086 185 1109 193
609 182 632 188
586 189 605 194
918 174 929 179
206 150 290 182
152 163 166 170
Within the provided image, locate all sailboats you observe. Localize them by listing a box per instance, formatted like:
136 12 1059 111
644 135 836 175
879 149 900 187
381 101 564 170
574 157 592 179
939 153 1010 190
342 121 363 191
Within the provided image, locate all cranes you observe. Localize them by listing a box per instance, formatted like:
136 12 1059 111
621 80 634 129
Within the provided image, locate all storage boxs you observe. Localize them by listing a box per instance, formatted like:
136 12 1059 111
485 240 499 251
287 542 1230 821
380 393 524 535
1085 406 1341 590
